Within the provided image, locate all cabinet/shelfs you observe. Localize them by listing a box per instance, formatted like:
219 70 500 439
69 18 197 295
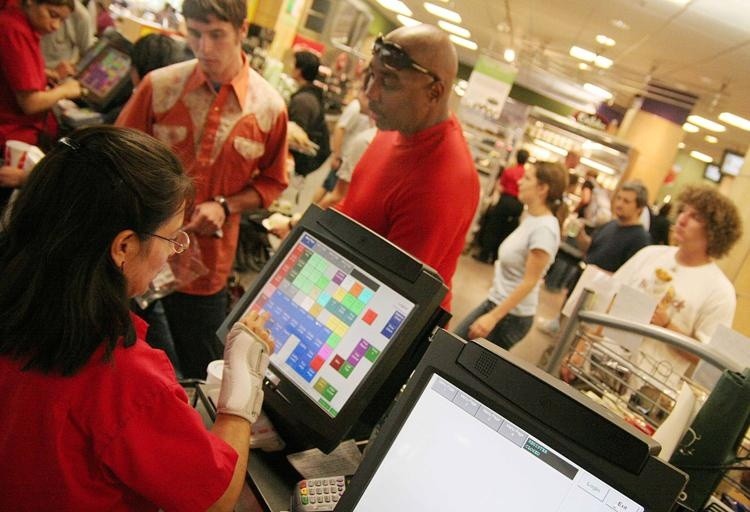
450 95 521 200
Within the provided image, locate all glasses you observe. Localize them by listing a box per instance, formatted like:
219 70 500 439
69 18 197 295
145 231 190 254
372 37 440 82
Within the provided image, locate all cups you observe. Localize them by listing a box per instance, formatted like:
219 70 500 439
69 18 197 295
566 218 582 238
4 140 44 175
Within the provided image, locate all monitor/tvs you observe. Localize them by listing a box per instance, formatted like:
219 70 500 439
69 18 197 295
77 41 133 111
332 326 690 512
719 149 745 177
210 201 450 452
703 163 723 184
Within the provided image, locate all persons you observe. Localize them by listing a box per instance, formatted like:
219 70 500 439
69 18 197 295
562 183 742 386
0 0 196 188
650 204 673 245
472 150 529 263
334 22 481 328
587 183 655 274
573 169 612 226
288 49 330 180
112 0 288 379
0 125 274 512
456 161 568 352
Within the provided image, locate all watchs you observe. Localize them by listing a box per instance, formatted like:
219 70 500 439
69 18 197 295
212 194 230 217
